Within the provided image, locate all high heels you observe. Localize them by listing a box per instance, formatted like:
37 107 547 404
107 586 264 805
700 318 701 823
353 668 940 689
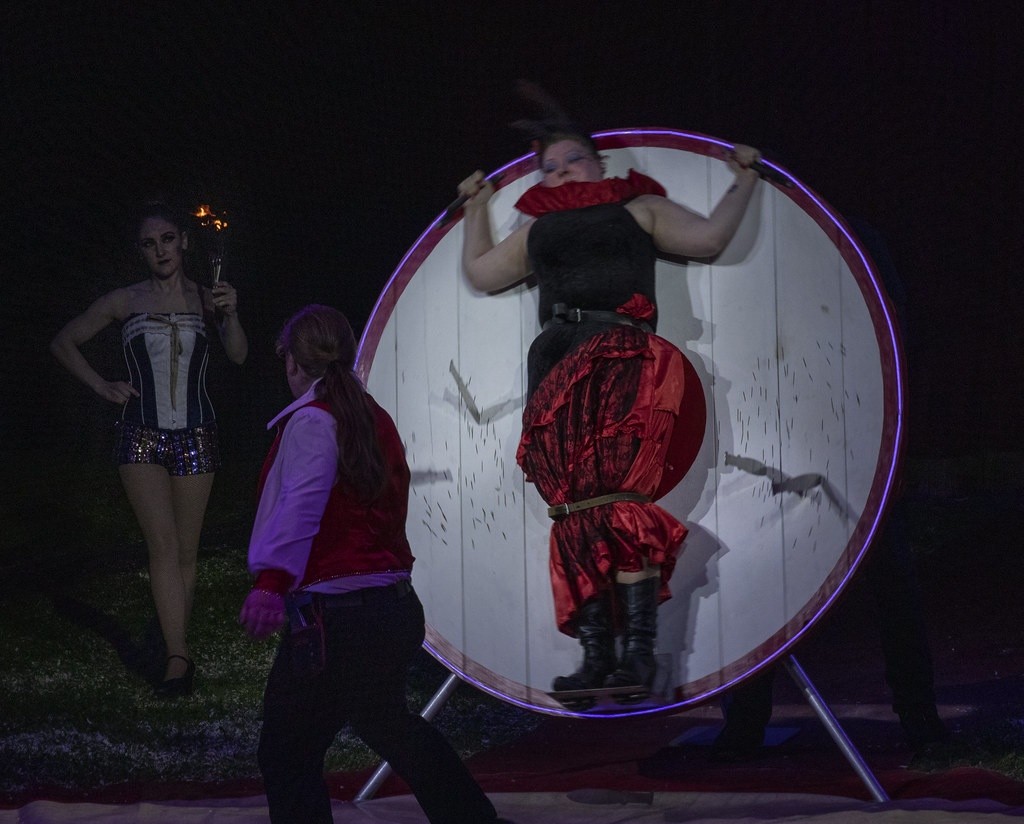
151 654 195 697
147 663 164 688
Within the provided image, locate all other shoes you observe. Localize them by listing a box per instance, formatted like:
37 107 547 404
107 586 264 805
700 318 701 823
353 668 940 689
898 705 945 744
711 718 764 759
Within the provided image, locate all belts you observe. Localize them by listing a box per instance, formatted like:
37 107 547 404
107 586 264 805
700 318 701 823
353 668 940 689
542 302 655 334
321 580 413 608
547 492 649 519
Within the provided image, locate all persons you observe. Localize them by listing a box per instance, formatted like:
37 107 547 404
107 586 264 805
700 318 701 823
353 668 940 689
238 304 520 824
50 207 252 699
457 79 765 704
709 511 950 770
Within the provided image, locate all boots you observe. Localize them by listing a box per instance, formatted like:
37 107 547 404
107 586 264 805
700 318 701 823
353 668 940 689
605 575 659 704
551 598 617 690
